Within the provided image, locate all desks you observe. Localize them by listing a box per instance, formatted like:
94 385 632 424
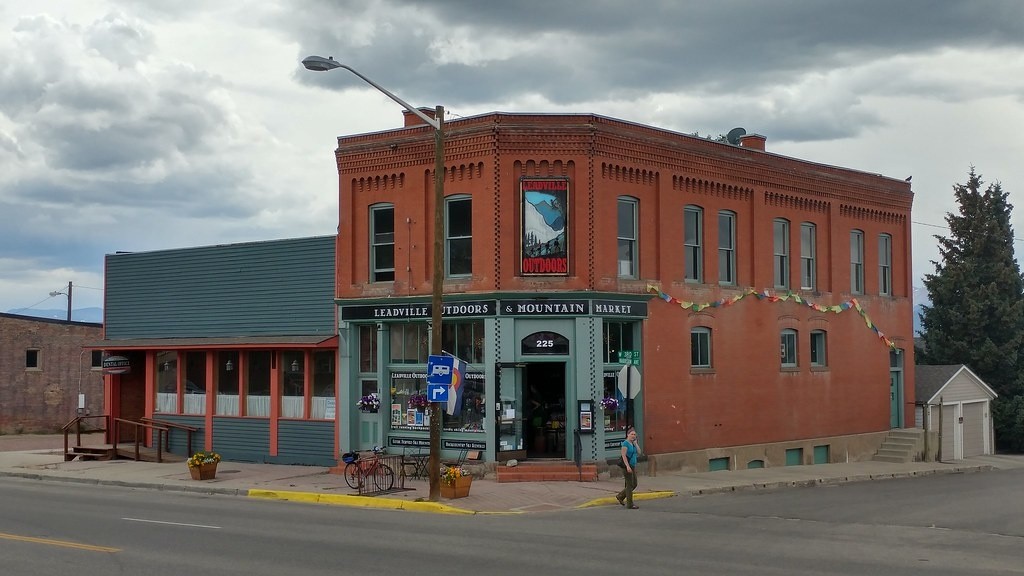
408 452 431 482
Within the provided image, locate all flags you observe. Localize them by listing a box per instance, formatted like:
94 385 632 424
441 354 466 417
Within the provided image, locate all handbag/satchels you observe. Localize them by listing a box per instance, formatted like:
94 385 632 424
617 454 631 468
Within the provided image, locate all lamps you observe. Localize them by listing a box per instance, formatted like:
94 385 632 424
291 360 299 371
226 360 233 371
164 361 170 371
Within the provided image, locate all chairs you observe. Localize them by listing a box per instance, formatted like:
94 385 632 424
444 446 469 468
397 446 421 480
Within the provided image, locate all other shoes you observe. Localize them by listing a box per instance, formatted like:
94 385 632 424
629 505 639 509
616 495 625 505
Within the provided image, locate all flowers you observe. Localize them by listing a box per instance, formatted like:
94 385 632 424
355 394 379 413
408 393 427 409
439 466 470 486
187 452 221 468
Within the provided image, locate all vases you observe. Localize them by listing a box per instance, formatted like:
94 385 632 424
440 475 470 498
189 463 216 480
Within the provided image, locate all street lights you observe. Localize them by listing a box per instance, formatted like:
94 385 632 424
49 281 72 321
301 56 445 501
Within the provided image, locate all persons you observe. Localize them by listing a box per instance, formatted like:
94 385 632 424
616 427 642 509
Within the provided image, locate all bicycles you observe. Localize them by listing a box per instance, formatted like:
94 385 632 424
344 446 394 491
